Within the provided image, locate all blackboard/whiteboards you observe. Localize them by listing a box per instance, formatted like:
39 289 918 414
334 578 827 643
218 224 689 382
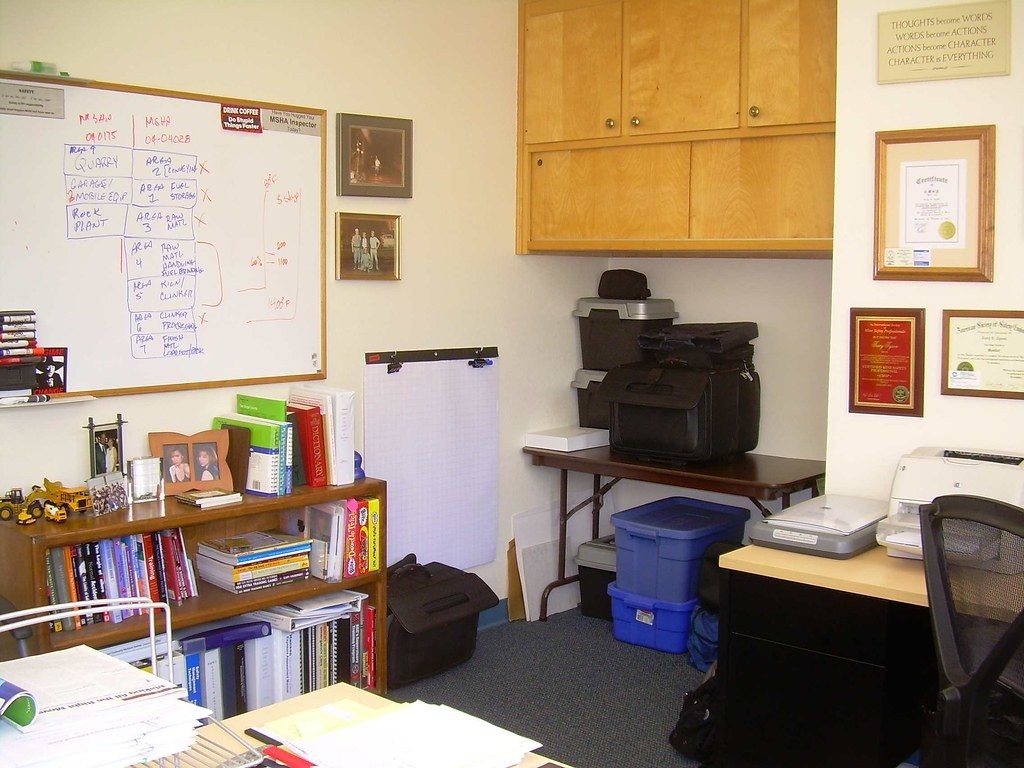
0 69 327 409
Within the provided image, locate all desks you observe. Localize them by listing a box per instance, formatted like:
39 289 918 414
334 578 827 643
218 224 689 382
122 679 572 768
712 539 1024 768
522 434 826 625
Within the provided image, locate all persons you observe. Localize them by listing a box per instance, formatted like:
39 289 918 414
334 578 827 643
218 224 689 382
351 228 381 272
374 156 380 176
198 446 218 481
95 432 117 474
92 482 127 515
169 446 191 482
36 355 64 389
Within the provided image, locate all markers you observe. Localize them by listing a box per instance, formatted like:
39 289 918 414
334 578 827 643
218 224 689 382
0 332 35 339
0 355 47 364
0 347 45 356
0 395 50 405
468 360 493 365
0 315 39 322
0 340 37 348
0 324 35 331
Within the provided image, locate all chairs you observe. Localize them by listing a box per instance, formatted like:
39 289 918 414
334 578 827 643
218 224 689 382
917 491 1024 768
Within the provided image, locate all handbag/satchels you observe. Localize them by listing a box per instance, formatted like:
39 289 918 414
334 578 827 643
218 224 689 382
387 561 500 687
669 674 919 768
637 322 759 352
596 362 760 463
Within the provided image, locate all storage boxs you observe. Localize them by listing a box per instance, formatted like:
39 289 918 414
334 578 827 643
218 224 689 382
572 530 618 619
571 293 680 430
608 495 750 657
386 552 501 691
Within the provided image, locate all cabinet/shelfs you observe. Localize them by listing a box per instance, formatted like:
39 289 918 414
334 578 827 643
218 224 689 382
516 1 839 259
1 476 388 720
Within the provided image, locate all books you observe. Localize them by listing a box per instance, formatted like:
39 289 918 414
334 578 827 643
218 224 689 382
98 589 376 726
211 381 354 496
0 644 214 768
174 487 242 508
525 426 609 452
44 527 199 633
196 528 313 595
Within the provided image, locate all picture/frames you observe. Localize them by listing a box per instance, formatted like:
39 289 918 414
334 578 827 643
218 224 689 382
84 412 128 480
149 429 233 496
337 112 414 199
335 210 402 281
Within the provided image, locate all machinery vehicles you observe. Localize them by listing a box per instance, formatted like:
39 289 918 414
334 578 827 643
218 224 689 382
0 478 92 525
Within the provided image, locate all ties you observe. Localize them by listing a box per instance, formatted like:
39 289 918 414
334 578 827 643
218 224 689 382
102 447 105 453
